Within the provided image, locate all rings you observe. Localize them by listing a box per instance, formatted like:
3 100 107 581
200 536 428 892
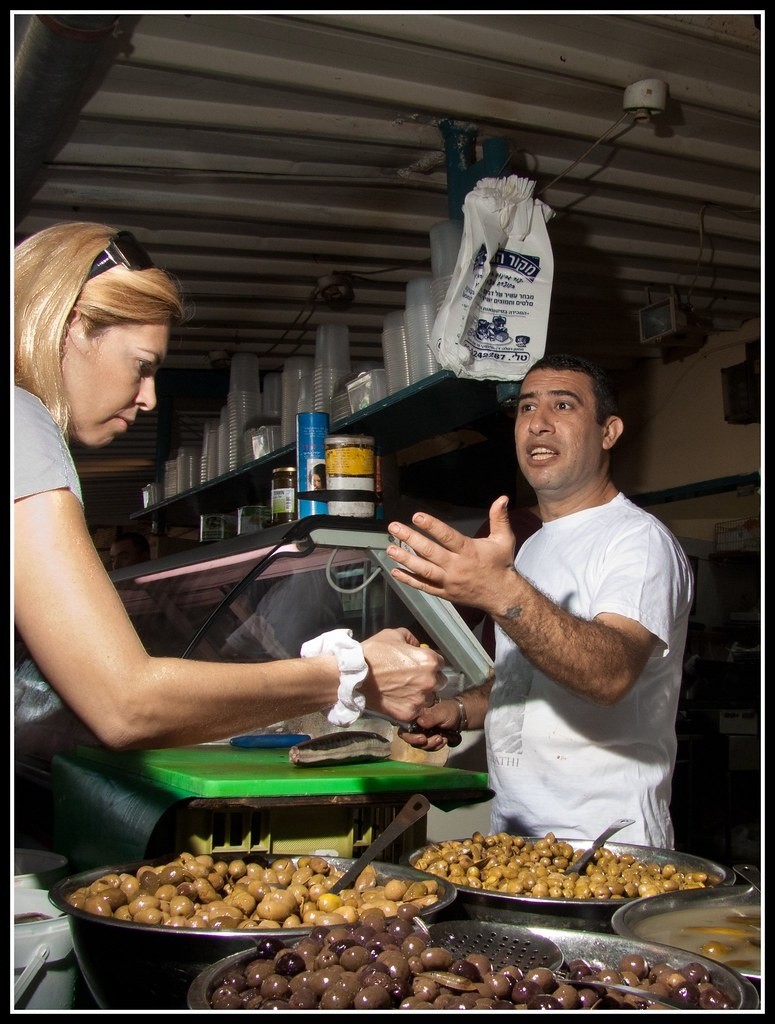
429 692 442 709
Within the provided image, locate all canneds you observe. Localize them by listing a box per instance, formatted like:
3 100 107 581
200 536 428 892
199 410 377 544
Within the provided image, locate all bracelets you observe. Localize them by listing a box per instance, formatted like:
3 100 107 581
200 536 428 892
301 627 371 728
453 698 466 733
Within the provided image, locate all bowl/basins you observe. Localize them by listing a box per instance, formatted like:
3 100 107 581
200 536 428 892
399 838 736 935
48 853 458 1012
187 927 760 1010
611 885 761 983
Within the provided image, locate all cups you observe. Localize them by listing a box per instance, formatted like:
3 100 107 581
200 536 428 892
175 220 461 495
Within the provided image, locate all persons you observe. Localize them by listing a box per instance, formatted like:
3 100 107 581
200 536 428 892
309 464 326 489
14 221 449 750
386 355 696 847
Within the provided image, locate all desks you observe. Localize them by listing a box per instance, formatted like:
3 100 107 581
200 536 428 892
15 747 494 864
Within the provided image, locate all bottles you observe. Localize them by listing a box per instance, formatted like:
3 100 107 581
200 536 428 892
271 468 297 524
324 435 376 519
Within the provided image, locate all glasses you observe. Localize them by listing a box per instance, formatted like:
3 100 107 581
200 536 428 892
83 230 156 282
